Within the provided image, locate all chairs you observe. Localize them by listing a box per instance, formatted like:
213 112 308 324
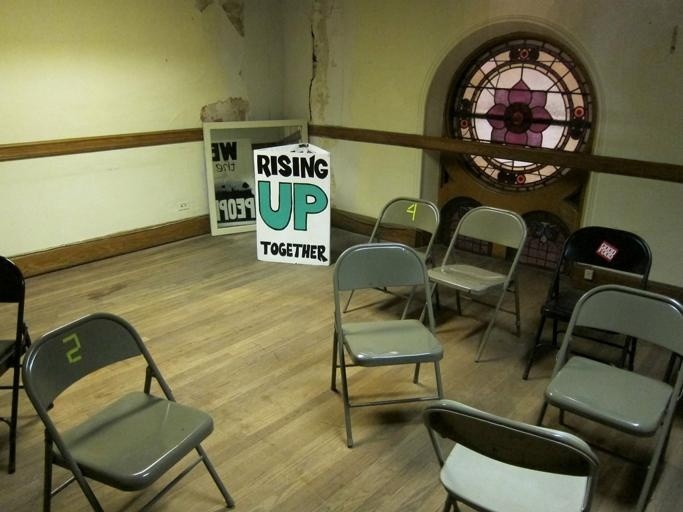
417 206 527 365
24 311 234 510
421 397 593 512
333 242 449 450
520 226 654 382
538 284 680 512
344 198 442 324
1 257 56 476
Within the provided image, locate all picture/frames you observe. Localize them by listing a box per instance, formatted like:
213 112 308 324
203 120 311 239
